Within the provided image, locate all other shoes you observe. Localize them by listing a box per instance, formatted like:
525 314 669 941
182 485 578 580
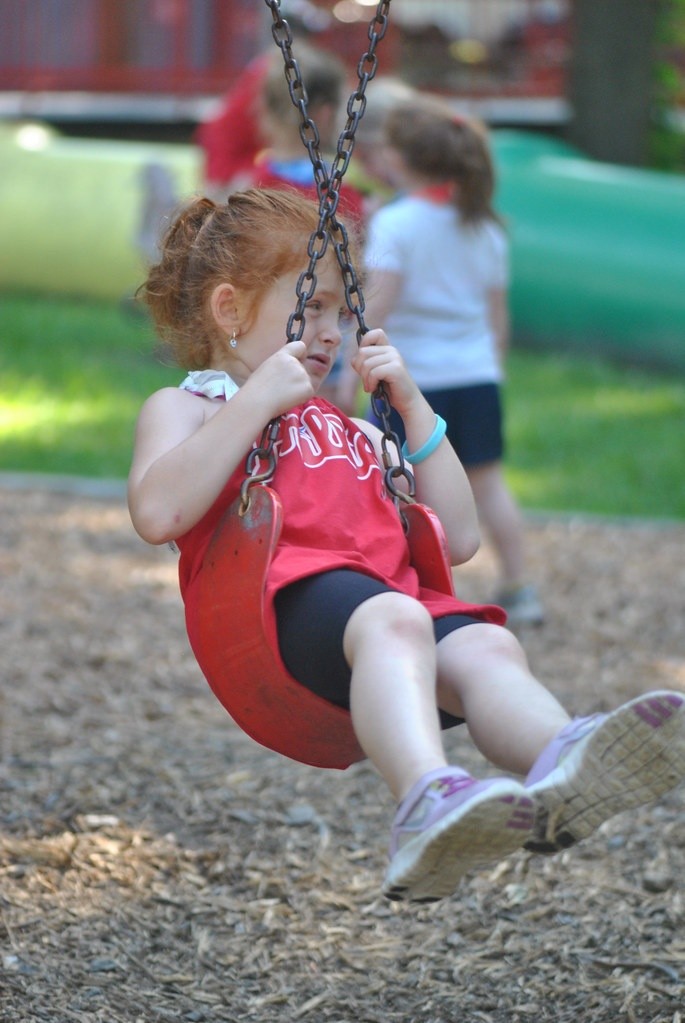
523 690 685 854
382 765 536 906
487 586 544 624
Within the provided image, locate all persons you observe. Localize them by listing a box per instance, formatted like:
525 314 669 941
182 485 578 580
127 185 685 906
228 46 371 402
337 98 544 625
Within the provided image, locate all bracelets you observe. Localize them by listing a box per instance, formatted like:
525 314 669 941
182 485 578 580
401 414 447 466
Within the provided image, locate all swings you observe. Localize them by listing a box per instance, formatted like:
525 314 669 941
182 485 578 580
180 1 454 770
